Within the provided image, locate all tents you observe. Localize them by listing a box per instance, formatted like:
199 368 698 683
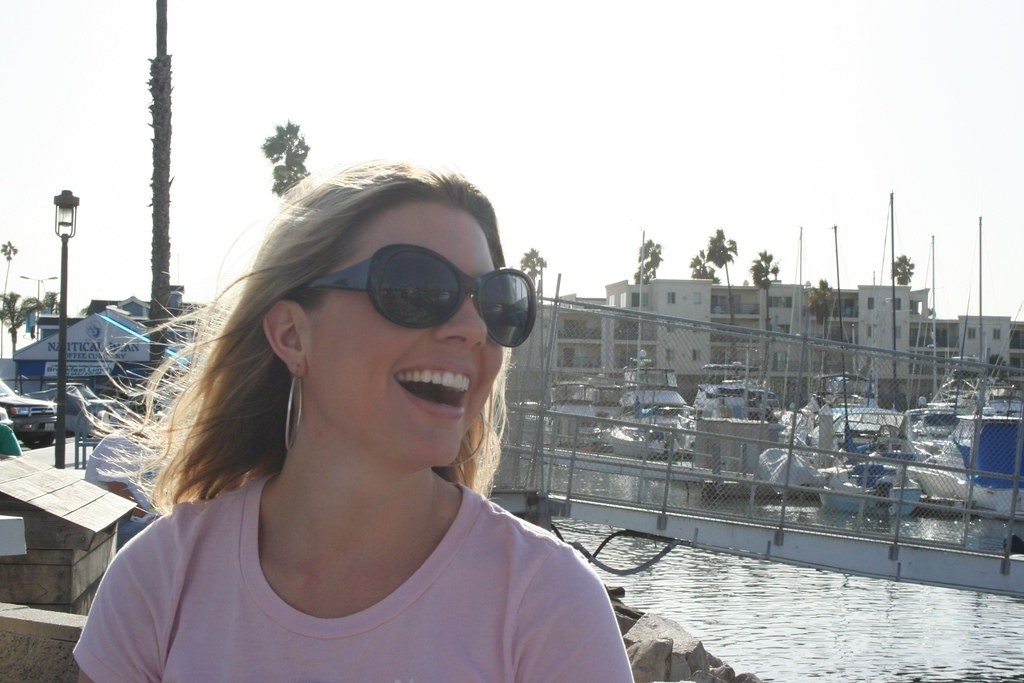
13 314 188 398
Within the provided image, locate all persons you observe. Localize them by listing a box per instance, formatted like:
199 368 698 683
71 160 636 683
0 407 22 457
85 395 166 546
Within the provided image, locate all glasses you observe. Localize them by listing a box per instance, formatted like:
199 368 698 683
288 243 537 348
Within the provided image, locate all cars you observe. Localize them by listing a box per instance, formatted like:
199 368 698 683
46 383 116 436
0 379 58 449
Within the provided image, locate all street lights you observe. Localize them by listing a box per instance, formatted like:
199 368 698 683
53 190 79 469
20 275 58 342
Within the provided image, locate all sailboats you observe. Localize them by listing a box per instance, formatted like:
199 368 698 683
509 190 1023 517
